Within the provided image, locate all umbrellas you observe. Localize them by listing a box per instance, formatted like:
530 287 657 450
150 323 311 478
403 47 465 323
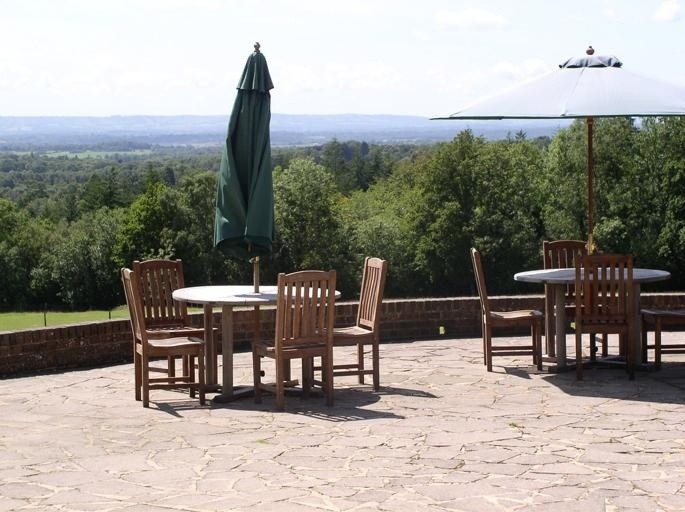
214 41 273 403
430 46 685 373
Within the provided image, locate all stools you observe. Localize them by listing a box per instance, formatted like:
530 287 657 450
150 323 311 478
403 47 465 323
641 308 685 367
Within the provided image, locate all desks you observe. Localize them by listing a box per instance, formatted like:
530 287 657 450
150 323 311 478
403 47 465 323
171 285 343 402
513 266 671 369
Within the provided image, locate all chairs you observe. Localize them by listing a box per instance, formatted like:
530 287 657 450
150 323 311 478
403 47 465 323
133 257 218 396
543 239 600 362
250 269 337 411
470 247 546 373
315 256 388 394
121 267 205 408
574 252 635 381
